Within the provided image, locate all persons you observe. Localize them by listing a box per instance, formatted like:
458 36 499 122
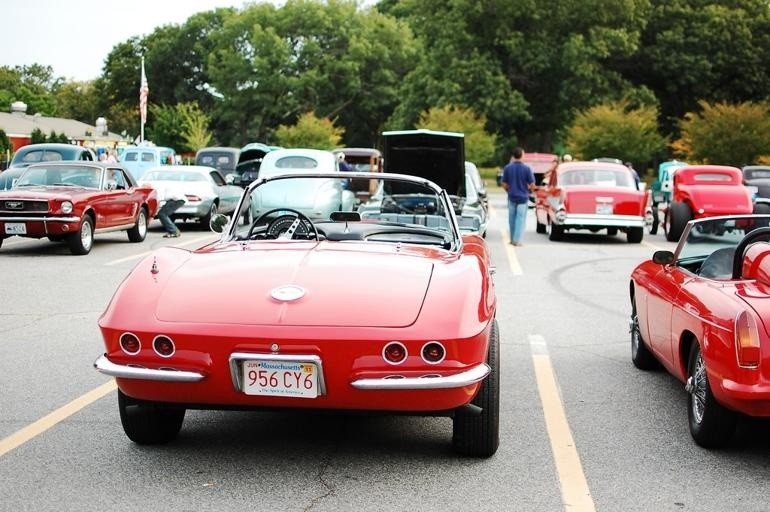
624 162 639 190
503 147 536 246
336 153 355 218
142 183 187 238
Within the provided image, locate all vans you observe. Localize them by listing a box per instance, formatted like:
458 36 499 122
196 147 283 184
334 148 383 201
121 148 175 168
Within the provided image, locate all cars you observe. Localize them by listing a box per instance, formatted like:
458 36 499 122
511 153 557 207
249 149 354 219
0 144 95 189
743 166 770 202
649 159 688 234
357 130 487 243
665 166 770 241
136 166 244 229
0 162 157 254
530 158 652 239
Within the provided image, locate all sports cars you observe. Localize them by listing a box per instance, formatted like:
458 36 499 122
631 216 770 447
94 172 500 457
